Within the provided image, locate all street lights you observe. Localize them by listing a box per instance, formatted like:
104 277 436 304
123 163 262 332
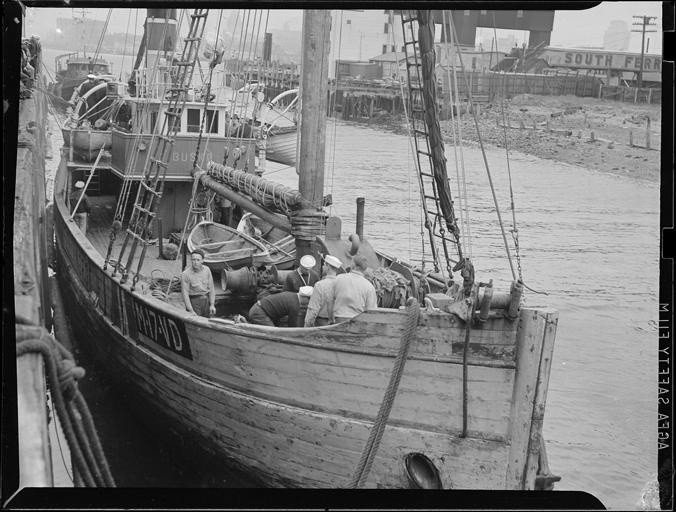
629 14 657 89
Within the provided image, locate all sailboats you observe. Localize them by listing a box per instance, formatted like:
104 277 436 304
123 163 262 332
47 0 560 493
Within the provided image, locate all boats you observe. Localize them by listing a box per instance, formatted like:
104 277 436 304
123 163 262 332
43 47 122 110
60 116 112 163
224 92 299 168
185 218 258 269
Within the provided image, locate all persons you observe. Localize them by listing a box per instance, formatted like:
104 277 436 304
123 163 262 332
248 253 377 327
79 74 106 126
225 114 253 138
20 35 41 97
180 248 216 318
72 180 91 235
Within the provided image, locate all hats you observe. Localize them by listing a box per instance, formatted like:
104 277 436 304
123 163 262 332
325 254 341 269
299 286 314 297
300 255 316 269
